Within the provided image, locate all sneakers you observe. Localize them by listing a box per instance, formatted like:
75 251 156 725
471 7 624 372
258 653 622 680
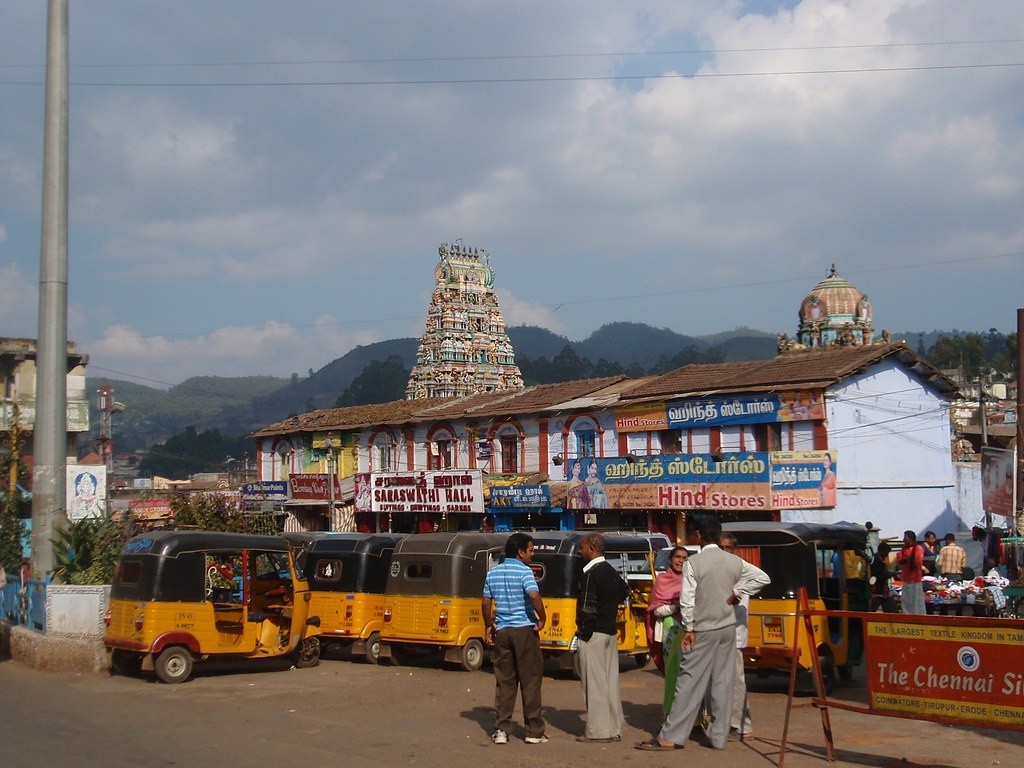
491 729 508 744
524 733 550 744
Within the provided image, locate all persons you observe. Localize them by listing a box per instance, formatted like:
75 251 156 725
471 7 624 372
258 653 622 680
567 459 592 509
818 454 836 507
780 390 823 420
831 541 902 613
717 532 755 742
896 530 927 615
934 533 966 615
644 546 690 716
971 558 1000 616
921 531 937 577
482 533 550 743
585 458 608 508
574 533 629 742
634 517 771 750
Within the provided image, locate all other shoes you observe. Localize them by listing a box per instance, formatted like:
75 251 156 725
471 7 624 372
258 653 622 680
576 735 622 743
728 730 754 741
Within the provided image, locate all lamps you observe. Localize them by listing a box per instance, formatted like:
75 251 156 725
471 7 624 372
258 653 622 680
552 452 587 467
711 446 745 462
625 449 663 464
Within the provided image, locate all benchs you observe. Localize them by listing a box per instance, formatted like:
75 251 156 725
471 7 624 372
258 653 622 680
210 588 266 625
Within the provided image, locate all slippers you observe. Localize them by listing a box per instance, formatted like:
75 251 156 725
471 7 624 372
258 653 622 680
634 739 676 751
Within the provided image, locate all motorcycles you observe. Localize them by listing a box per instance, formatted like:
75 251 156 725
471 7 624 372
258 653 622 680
273 529 674 673
702 521 877 682
99 529 321 683
